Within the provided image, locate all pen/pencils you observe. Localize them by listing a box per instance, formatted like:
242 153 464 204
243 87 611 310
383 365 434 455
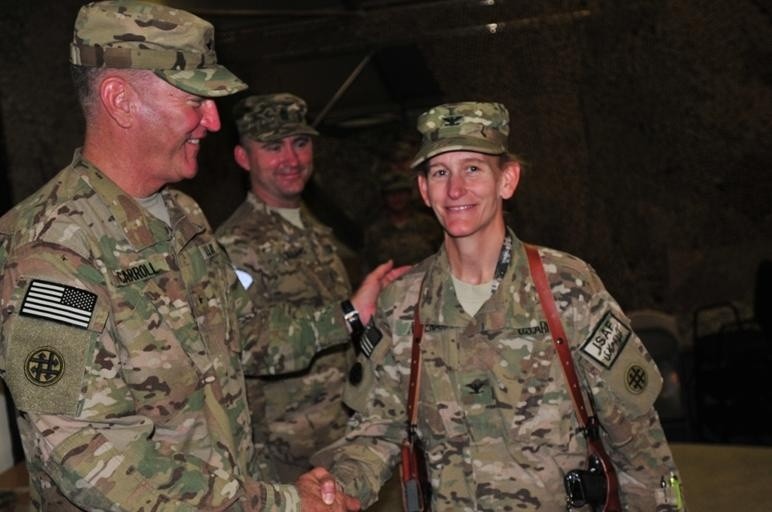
662 474 685 510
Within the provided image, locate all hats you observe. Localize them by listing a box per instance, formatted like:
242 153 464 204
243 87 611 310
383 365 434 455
67 0 249 98
408 101 511 172
232 93 321 143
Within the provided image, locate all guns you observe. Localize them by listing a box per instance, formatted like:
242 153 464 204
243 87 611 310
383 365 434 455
564 452 609 512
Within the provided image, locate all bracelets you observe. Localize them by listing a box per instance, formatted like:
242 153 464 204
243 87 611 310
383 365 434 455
342 296 366 333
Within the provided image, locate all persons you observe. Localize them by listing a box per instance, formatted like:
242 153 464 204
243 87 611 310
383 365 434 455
309 99 689 512
216 94 403 512
362 167 441 273
0 2 363 512
